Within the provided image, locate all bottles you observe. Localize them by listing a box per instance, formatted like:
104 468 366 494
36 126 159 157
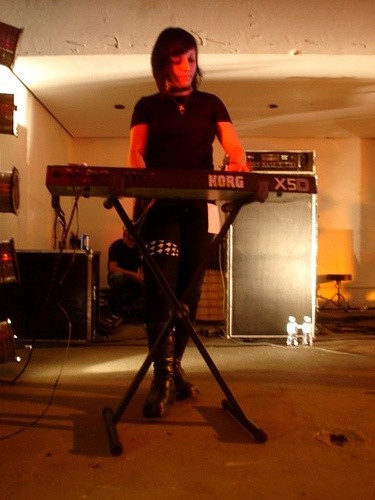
302 316 313 346
287 315 298 346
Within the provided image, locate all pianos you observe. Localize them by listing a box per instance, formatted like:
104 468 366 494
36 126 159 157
45 164 270 457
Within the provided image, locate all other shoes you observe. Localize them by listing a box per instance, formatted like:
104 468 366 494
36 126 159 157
106 306 130 326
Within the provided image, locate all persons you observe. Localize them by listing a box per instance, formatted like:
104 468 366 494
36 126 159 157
107 224 148 324
129 26 248 418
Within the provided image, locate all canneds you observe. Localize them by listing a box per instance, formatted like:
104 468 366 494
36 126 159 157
80 235 89 251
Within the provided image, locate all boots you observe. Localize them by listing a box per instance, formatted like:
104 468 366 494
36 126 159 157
143 322 176 418
174 315 199 400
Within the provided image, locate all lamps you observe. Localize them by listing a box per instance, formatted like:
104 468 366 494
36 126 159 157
0 21 24 69
0 166 20 215
0 237 21 286
0 93 17 136
0 317 21 363
